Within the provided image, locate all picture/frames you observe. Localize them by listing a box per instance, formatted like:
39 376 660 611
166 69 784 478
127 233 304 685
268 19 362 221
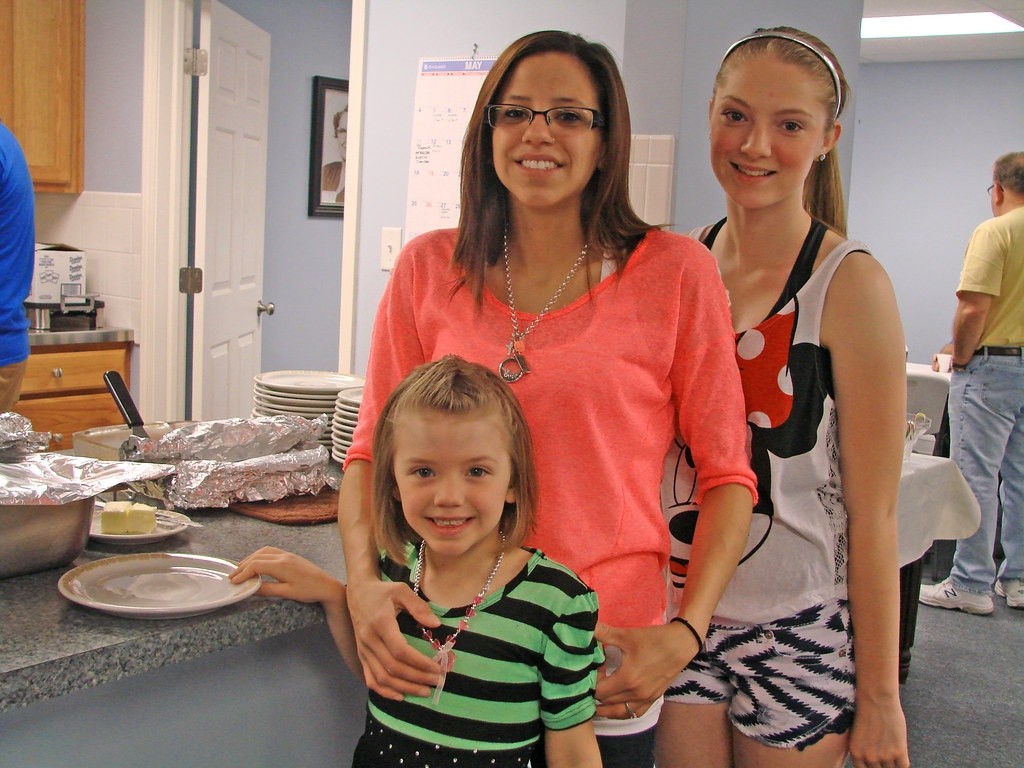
308 75 348 219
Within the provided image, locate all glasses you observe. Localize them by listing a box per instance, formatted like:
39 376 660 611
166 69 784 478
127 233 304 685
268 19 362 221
488 104 605 130
987 184 1004 195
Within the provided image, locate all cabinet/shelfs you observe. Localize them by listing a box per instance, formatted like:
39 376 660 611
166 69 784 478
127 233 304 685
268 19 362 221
0 0 86 196
13 341 132 452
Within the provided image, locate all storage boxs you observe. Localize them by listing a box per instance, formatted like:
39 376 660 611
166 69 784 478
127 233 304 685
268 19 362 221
24 241 86 304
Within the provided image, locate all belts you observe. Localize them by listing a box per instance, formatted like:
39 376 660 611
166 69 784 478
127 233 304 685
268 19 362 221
973 346 1021 356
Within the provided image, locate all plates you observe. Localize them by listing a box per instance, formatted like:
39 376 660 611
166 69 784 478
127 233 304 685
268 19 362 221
89 509 192 545
251 370 365 463
58 551 263 620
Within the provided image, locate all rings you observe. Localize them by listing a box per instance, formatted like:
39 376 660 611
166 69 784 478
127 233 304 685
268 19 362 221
624 702 637 719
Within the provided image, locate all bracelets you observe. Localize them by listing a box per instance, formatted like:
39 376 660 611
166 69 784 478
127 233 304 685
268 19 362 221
670 617 703 655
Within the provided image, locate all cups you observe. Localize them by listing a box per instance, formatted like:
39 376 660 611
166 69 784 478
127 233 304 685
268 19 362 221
937 354 951 373
903 413 932 463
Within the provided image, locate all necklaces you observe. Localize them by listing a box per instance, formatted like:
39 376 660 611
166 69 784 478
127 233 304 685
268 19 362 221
413 530 507 706
499 210 594 383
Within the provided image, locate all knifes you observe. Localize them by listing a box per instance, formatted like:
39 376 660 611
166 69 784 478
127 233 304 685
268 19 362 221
94 500 204 528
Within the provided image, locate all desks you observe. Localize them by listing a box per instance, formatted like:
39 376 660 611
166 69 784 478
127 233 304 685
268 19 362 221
896 452 981 685
906 359 953 455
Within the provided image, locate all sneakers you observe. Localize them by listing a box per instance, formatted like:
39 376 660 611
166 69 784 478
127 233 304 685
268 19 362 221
919 576 994 614
995 579 1024 606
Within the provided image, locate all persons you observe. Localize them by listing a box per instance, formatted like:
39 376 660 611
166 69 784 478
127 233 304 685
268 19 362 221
229 353 607 768
932 339 954 371
0 120 37 410
337 29 758 768
918 151 1024 614
653 25 911 768
